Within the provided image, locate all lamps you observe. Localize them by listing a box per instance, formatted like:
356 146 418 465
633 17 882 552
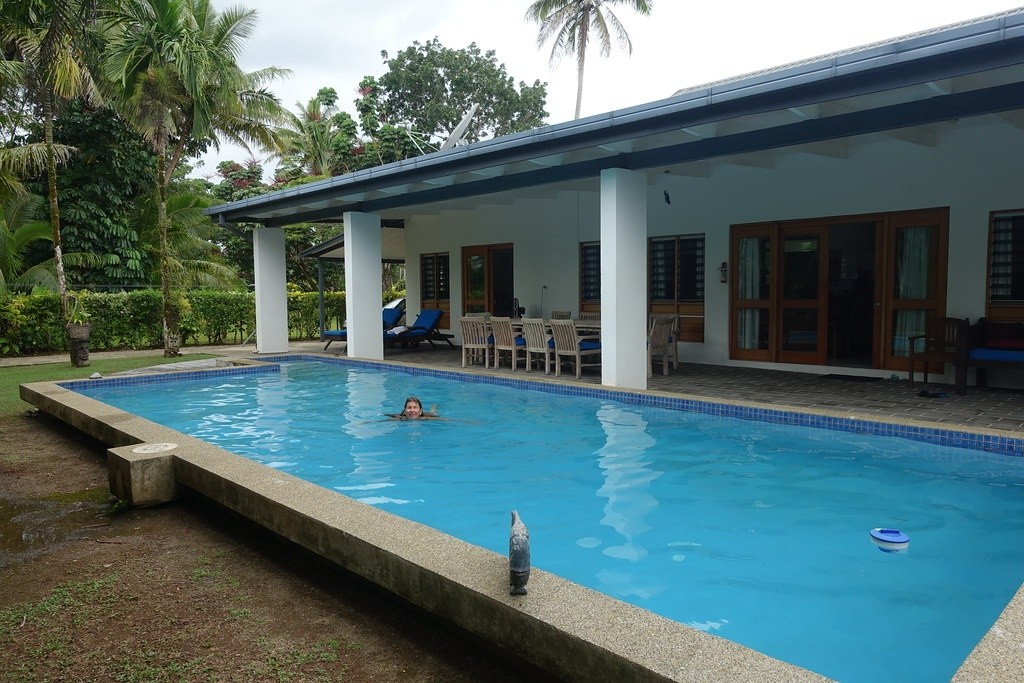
720 262 728 283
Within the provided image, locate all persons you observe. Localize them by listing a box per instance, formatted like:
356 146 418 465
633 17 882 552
400 396 425 419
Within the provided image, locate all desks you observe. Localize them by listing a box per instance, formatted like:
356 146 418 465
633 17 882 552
477 318 602 374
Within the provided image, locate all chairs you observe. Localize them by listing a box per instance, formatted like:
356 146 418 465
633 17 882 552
908 317 970 393
383 308 456 357
458 310 679 379
324 297 406 353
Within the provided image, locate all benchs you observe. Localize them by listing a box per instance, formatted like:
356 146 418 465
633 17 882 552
948 317 1024 395
